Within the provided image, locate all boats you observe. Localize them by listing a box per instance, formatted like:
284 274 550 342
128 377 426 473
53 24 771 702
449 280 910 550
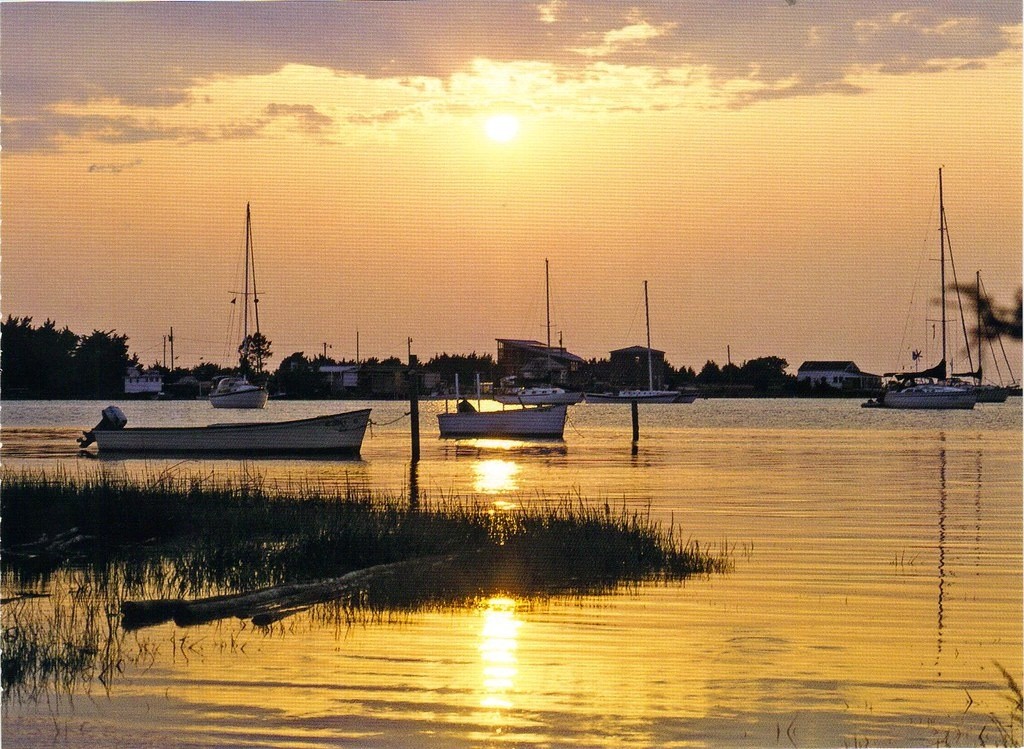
434 397 569 438
75 405 371 456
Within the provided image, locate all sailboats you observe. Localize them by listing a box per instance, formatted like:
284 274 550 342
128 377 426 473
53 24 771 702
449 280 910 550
205 201 270 411
859 164 1023 410
492 257 587 406
583 279 681 406
